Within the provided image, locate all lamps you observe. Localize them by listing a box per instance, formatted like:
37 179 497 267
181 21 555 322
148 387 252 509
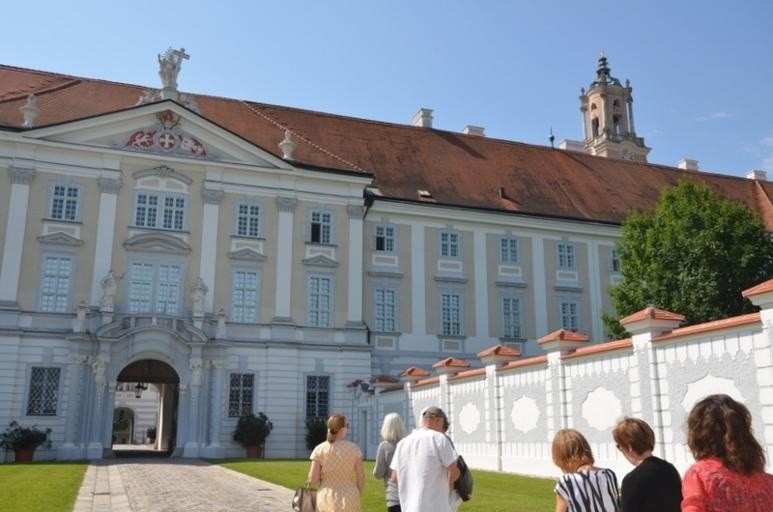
131 381 149 400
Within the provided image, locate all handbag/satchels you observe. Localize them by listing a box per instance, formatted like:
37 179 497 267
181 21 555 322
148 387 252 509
292 480 318 512
454 454 473 502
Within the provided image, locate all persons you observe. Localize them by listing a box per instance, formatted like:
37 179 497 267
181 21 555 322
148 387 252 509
190 277 208 313
308 415 363 512
157 53 182 87
679 394 772 511
551 429 619 512
99 269 118 308
372 413 405 511
612 416 682 512
389 406 460 512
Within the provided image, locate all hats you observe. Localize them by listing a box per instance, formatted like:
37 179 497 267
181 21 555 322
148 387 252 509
422 406 447 431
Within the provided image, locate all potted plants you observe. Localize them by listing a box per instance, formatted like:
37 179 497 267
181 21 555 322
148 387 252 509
0 418 53 463
233 412 274 460
144 427 155 443
